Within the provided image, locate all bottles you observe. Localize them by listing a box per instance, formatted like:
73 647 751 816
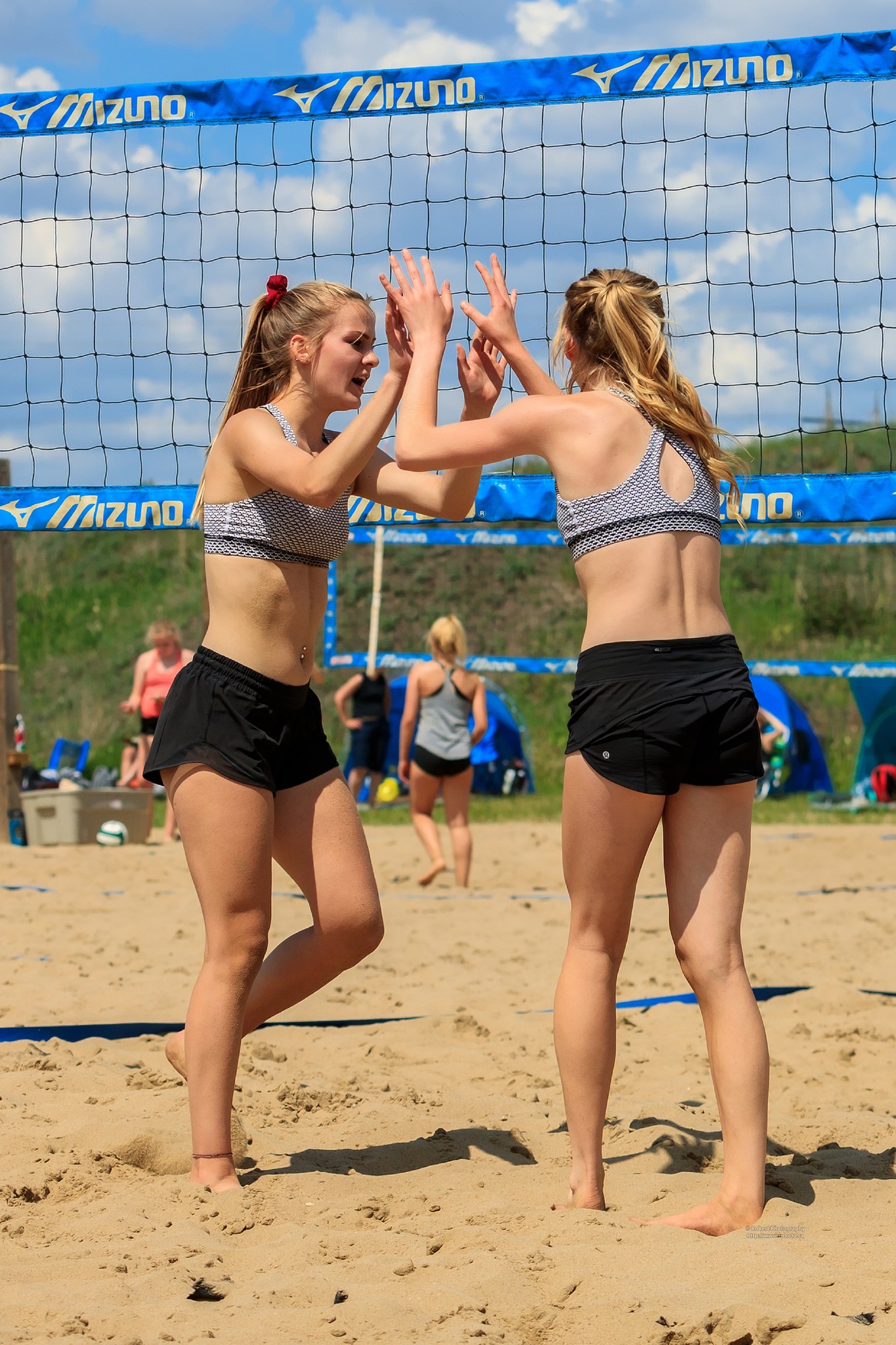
14 714 26 753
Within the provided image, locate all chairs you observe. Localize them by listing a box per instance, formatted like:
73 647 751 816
47 736 90 772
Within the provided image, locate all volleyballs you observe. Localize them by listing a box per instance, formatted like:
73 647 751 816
96 818 128 846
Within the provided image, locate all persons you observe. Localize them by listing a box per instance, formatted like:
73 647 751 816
333 666 390 809
142 275 508 1194
756 706 786 770
379 248 771 1237
397 614 487 890
113 619 197 840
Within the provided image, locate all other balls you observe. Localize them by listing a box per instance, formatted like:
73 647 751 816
375 774 400 801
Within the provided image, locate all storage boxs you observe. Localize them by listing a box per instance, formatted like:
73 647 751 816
20 785 154 845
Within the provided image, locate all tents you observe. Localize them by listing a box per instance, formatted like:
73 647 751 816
751 676 834 801
341 674 537 799
